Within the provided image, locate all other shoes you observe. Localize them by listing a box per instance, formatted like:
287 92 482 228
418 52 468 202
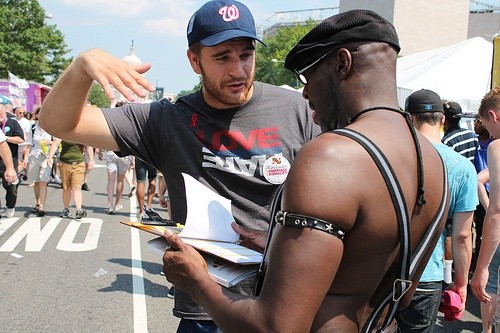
49 176 52 183
107 207 115 214
115 204 123 211
5 206 15 218
60 208 69 217
81 183 90 191
75 209 87 219
55 175 59 181
136 211 145 218
128 185 136 197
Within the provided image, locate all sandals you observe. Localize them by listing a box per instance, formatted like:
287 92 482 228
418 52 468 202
31 204 46 217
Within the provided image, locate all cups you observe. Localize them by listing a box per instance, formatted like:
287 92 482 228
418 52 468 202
39 140 48 155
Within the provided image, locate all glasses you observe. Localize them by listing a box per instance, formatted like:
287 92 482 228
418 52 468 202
293 46 359 85
19 112 24 114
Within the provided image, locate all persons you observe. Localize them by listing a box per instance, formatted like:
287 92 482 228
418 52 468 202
405 89 500 333
0 102 170 220
162 9 450 333
37 0 323 333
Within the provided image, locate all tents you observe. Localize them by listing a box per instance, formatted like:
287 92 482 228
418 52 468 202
388 35 494 133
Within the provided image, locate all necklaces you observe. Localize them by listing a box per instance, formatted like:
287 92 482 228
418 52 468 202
346 104 426 206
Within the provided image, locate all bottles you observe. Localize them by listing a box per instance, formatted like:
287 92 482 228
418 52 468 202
4 173 19 185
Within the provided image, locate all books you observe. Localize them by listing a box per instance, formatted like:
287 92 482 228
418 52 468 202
146 235 258 289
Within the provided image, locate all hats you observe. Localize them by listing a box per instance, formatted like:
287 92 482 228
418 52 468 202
438 290 463 321
404 88 444 114
441 99 462 119
283 8 401 72
186 0 267 48
